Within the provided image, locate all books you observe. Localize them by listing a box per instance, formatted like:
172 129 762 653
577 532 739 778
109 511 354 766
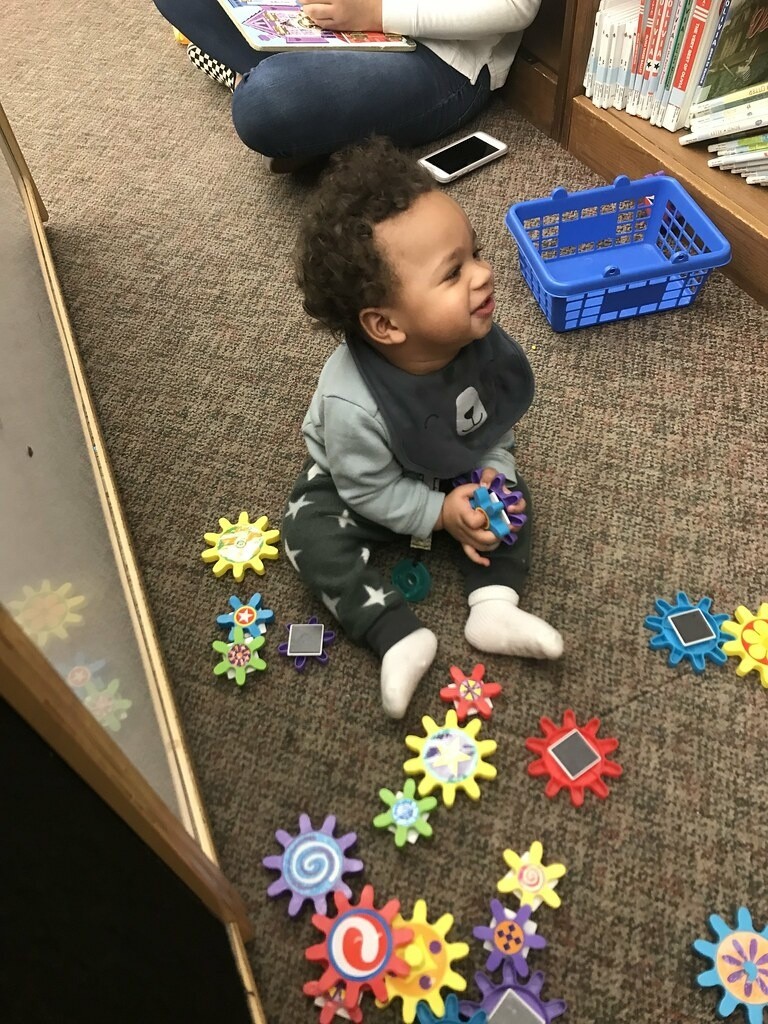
582 0 767 188
216 0 416 53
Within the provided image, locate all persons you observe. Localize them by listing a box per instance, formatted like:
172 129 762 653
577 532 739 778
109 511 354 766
150 0 541 180
278 137 565 722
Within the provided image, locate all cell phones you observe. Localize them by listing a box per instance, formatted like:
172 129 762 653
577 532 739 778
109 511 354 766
416 130 508 184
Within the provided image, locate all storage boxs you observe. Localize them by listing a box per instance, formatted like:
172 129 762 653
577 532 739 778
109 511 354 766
504 174 733 334
